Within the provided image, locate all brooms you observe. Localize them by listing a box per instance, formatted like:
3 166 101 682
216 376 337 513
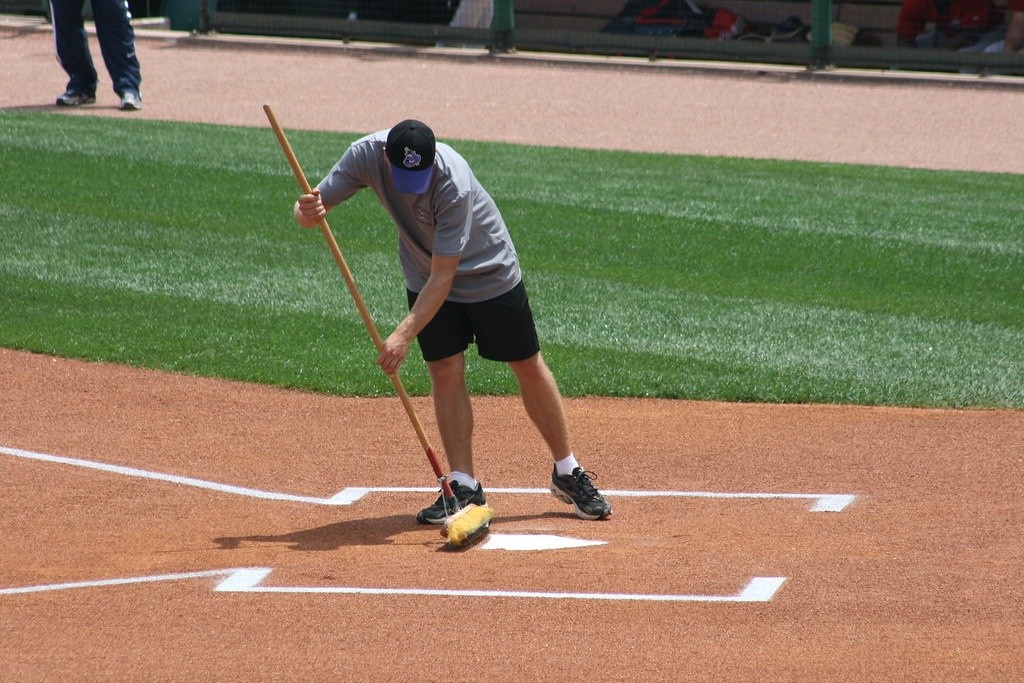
262 101 494 550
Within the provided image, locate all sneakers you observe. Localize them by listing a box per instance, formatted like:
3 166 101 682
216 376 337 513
415 479 487 524
550 463 613 520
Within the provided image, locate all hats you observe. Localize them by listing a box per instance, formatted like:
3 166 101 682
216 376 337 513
387 119 436 194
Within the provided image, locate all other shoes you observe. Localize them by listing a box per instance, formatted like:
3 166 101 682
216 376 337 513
56 88 96 106
119 93 141 110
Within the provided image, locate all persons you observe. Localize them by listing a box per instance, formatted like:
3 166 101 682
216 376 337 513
292 120 612 525
49 0 142 109
